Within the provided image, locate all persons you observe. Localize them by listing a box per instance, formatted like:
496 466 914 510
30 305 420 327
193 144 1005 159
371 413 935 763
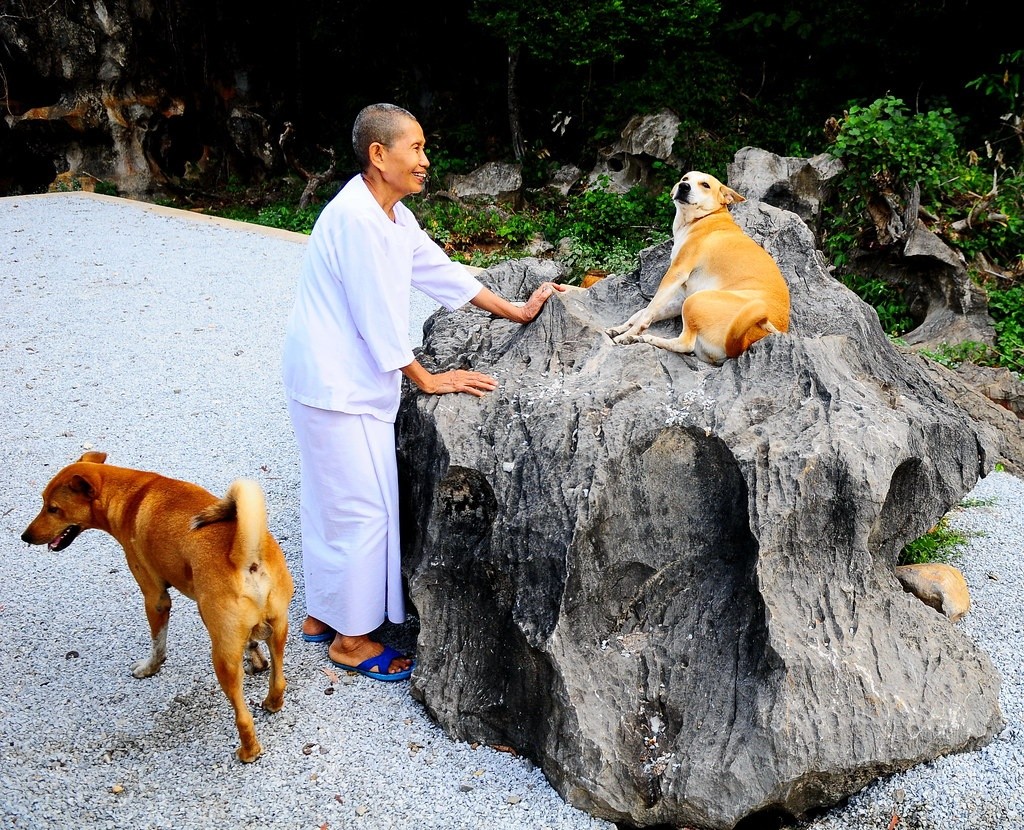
280 103 566 682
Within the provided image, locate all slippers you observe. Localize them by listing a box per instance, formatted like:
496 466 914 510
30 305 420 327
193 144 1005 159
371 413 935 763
302 629 337 643
331 644 418 682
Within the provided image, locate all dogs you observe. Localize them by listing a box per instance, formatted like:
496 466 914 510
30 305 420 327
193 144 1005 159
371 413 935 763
605 170 791 366
20 450 295 764
